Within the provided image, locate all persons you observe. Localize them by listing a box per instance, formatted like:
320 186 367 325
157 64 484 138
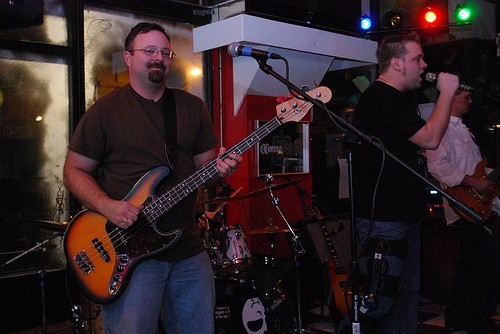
62 22 243 334
349 28 459 334
427 57 500 334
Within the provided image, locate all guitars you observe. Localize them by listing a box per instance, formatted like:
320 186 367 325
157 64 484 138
445 161 499 221
62 85 333 306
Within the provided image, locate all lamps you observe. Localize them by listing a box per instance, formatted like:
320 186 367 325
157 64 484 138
454 3 473 25
357 13 375 32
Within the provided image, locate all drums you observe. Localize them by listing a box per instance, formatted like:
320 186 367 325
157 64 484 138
212 257 282 306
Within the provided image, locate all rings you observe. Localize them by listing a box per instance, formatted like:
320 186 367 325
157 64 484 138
122 219 128 223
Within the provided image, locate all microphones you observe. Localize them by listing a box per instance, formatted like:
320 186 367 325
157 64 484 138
26 217 70 231
425 73 475 90
227 42 281 59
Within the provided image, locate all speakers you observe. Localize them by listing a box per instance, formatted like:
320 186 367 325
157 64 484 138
293 213 369 277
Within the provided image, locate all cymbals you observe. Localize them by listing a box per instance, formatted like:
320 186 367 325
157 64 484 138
30 219 64 231
245 226 297 235
197 195 246 204
243 179 301 197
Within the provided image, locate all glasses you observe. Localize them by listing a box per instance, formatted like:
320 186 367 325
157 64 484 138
128 47 176 59
